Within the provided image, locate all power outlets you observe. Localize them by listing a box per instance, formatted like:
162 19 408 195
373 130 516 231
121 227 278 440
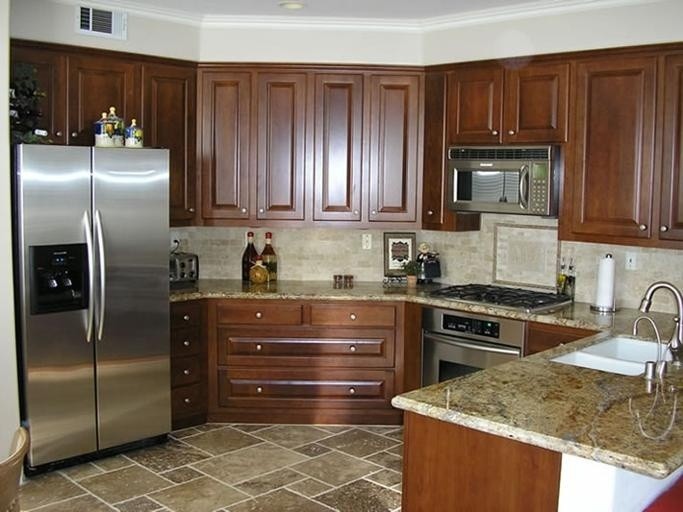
171 232 179 250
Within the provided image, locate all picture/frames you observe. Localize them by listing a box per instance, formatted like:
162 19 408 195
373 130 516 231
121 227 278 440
383 232 417 278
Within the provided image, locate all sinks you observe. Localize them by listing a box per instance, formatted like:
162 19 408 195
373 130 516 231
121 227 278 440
550 352 646 378
583 335 673 364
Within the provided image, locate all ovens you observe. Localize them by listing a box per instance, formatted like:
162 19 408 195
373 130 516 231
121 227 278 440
423 331 522 385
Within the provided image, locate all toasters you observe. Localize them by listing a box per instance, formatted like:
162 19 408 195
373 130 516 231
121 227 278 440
169 252 201 283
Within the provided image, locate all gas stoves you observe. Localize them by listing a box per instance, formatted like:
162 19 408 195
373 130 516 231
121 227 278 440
420 284 569 351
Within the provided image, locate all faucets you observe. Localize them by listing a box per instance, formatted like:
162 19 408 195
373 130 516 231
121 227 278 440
633 316 666 376
639 281 683 366
635 386 677 441
627 383 659 422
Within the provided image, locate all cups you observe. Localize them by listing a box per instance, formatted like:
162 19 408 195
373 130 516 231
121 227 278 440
334 275 354 282
334 282 353 289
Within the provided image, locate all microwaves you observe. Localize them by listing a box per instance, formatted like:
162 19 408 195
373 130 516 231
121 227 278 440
446 145 561 215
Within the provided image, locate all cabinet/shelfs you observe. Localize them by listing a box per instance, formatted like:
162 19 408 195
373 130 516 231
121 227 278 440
310 70 421 223
138 62 197 220
171 300 208 429
207 298 423 425
446 62 574 144
198 69 310 220
7 46 137 144
569 51 683 241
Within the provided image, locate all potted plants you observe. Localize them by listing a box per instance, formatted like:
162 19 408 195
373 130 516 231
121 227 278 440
403 262 421 287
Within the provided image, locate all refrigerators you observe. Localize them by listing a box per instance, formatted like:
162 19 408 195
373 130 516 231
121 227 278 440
12 144 172 478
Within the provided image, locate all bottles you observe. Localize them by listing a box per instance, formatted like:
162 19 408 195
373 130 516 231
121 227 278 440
93 106 145 148
242 232 278 282
557 256 575 298
242 282 278 293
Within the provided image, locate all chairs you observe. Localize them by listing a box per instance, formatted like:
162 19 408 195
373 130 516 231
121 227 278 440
0 426 30 512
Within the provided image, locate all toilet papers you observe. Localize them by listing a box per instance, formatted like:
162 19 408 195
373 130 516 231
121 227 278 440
596 257 615 308
